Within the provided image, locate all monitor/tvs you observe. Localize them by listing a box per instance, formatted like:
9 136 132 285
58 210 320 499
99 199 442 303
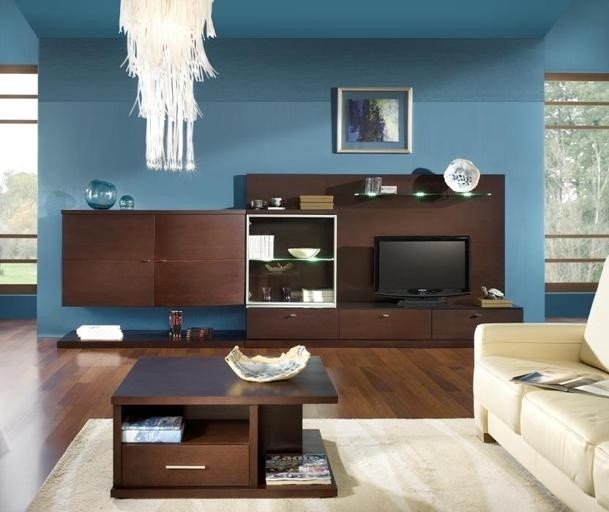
372 233 472 308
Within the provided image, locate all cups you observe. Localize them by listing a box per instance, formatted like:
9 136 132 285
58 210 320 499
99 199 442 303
363 177 383 194
270 197 288 207
281 287 291 302
251 200 267 210
261 287 273 301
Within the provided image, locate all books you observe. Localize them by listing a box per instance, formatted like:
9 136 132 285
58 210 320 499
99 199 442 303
262 452 334 486
249 235 274 259
509 365 608 399
76 324 125 342
303 289 334 302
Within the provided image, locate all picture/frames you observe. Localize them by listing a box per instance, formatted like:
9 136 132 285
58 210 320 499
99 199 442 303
334 85 414 154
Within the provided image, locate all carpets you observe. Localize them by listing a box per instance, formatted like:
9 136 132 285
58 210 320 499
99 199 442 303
23 416 568 511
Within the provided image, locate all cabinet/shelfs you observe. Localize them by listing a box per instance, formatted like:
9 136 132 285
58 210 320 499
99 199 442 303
60 208 247 307
244 303 525 347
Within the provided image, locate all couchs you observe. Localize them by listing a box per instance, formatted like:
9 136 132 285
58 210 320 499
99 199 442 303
472 257 609 511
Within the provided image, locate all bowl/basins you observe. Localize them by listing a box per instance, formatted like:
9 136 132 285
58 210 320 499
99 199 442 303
287 247 322 259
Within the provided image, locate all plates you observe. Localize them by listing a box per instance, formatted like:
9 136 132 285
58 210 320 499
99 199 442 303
443 158 481 193
